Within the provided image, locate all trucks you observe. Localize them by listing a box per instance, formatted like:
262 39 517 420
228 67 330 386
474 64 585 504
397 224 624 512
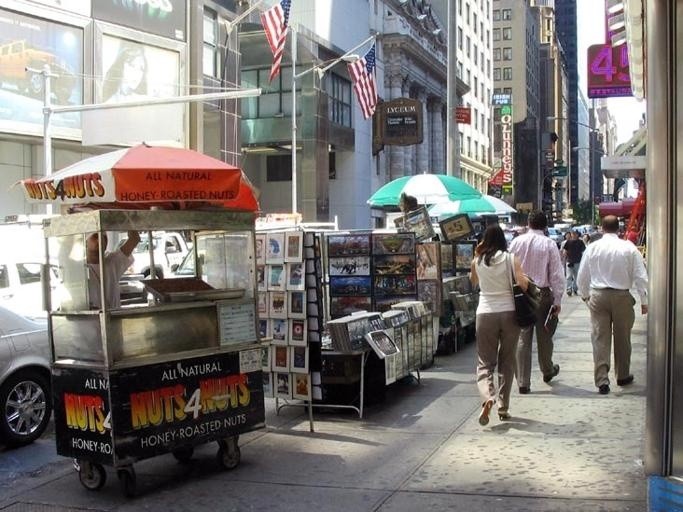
0 213 63 266
172 240 209 283
119 232 191 281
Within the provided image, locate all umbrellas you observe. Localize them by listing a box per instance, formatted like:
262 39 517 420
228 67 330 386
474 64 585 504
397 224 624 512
366 171 482 208
10 141 261 307
425 194 517 219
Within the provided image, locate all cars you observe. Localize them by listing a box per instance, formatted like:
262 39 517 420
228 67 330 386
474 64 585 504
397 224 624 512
0 306 52 447
546 223 592 245
0 258 69 316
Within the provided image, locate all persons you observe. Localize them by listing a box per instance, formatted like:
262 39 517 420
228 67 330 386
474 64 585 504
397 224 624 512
101 43 158 105
559 229 591 297
576 214 649 394
57 206 141 312
508 210 566 394
469 226 528 426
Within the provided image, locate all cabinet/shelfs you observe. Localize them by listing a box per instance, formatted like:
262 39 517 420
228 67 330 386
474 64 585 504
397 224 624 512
242 226 481 413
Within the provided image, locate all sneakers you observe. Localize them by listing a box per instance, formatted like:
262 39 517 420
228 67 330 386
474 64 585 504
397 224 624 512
543 365 559 382
519 386 531 393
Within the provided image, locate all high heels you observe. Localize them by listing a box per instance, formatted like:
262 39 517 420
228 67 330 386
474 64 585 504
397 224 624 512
478 399 493 425
497 409 510 420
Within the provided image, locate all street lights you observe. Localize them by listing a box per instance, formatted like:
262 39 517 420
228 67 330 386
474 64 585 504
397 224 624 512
546 115 611 207
540 169 560 213
570 145 610 202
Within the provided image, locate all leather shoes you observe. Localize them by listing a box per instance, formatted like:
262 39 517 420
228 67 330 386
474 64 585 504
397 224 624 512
599 384 610 393
617 374 633 386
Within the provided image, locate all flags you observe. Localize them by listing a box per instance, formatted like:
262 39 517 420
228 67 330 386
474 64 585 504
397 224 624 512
346 36 378 120
259 0 291 84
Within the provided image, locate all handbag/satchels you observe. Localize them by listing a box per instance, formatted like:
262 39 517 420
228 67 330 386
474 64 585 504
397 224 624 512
512 285 539 328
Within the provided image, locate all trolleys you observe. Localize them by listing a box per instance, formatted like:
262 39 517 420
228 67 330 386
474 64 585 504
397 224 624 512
41 207 265 498
